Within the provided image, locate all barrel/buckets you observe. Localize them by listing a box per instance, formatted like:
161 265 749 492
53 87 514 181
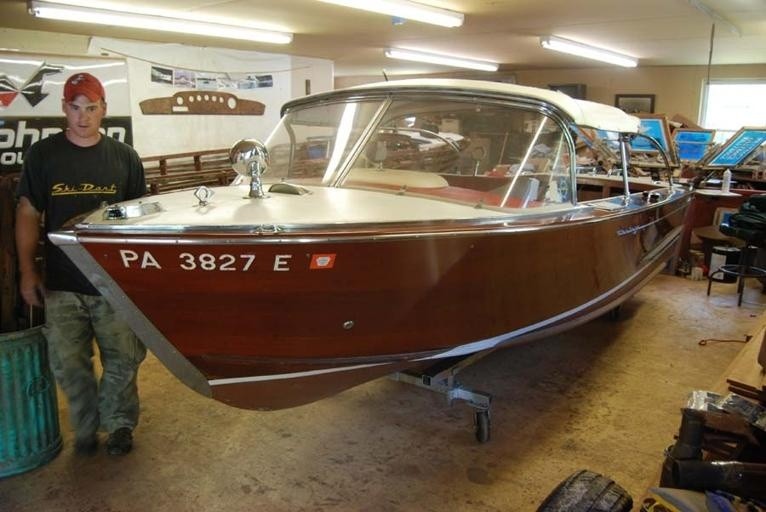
0 326 62 479
709 246 740 282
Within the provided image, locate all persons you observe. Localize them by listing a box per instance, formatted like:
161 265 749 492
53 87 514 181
14 72 148 456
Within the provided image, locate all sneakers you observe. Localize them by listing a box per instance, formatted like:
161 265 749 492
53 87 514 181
107 427 133 455
72 439 98 454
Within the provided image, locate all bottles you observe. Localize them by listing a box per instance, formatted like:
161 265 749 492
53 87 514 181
722 166 732 195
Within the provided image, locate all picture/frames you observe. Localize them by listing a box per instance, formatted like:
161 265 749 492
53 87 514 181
615 93 656 114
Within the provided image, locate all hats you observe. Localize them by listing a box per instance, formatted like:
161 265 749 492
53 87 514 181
64 72 104 106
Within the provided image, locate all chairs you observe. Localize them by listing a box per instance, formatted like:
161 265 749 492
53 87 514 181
705 193 766 308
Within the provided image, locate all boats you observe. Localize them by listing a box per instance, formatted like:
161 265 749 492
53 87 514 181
47 80 698 411
